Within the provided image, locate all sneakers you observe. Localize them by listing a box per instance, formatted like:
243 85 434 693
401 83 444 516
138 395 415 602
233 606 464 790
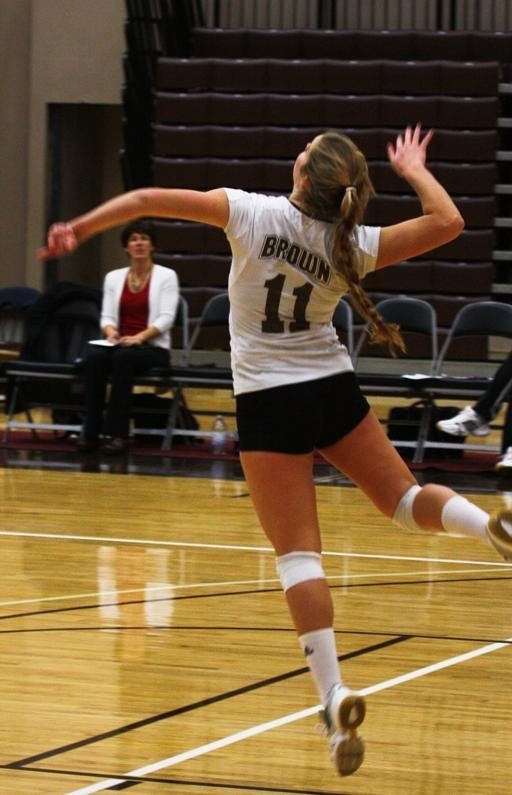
486 507 512 565
103 438 130 457
77 436 100 450
495 446 512 474
437 404 491 436
318 684 367 777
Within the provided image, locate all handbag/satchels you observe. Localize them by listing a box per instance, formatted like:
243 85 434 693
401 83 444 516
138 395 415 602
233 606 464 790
387 399 467 460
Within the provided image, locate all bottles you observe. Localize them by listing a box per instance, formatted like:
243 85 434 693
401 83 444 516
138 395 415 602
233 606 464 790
211 414 239 455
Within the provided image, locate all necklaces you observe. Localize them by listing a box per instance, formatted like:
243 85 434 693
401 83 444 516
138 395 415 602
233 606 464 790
129 264 152 292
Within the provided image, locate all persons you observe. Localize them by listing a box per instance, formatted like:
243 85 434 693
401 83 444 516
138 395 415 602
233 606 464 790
77 218 182 476
35 118 511 778
433 350 511 476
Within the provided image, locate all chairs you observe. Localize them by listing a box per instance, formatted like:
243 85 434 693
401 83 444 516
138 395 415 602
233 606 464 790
2 287 48 450
144 295 247 462
90 297 191 460
5 289 112 454
349 298 438 464
416 301 512 464
334 301 355 352
